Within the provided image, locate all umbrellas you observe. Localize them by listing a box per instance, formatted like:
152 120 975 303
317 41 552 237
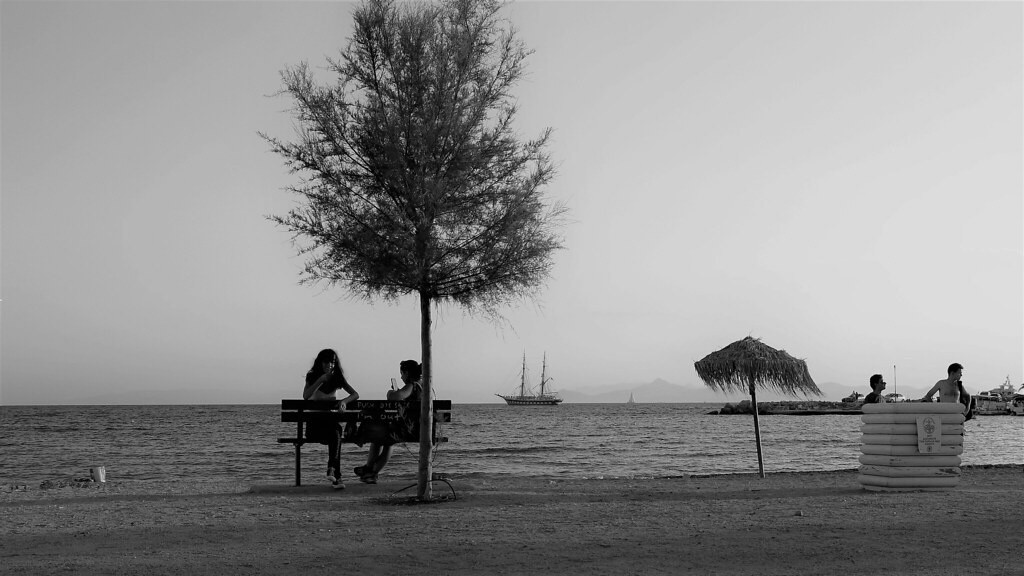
692 336 826 480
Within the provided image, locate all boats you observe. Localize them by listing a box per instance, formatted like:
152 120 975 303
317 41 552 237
494 348 564 405
1010 395 1024 415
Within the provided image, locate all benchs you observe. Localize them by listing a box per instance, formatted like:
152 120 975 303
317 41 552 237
277 398 452 488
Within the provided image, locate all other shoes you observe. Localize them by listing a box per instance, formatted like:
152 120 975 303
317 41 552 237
353 464 379 484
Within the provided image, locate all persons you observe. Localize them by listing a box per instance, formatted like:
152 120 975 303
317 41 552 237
864 373 887 402
302 347 359 490
922 362 972 417
353 359 436 484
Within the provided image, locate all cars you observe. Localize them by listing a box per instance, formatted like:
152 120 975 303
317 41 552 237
931 397 937 402
884 393 906 403
841 393 865 403
979 391 1002 403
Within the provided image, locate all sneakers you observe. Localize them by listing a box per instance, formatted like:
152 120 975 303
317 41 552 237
326 466 346 489
